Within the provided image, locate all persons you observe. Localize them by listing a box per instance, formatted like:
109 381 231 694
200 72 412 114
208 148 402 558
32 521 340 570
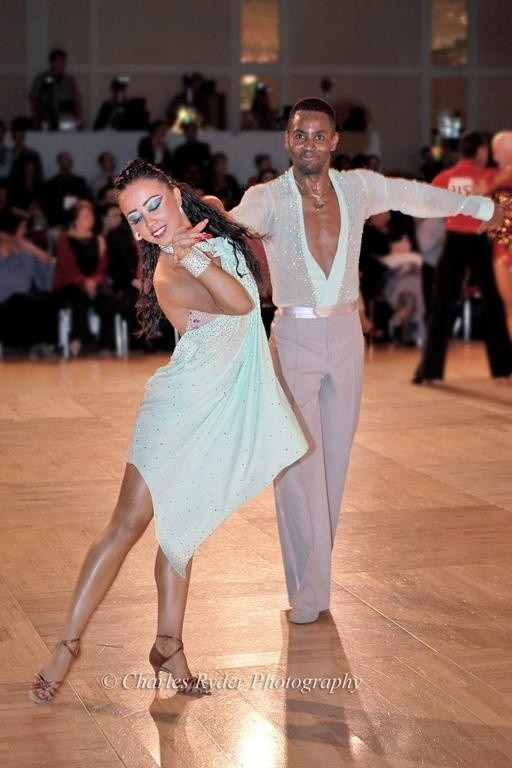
0 43 512 389
28 157 311 705
224 94 512 627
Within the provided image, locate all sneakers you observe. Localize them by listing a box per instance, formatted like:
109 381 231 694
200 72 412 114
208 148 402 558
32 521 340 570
290 609 319 623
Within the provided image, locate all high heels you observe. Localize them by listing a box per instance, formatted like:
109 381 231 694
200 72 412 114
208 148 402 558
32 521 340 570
150 634 205 693
29 637 81 704
